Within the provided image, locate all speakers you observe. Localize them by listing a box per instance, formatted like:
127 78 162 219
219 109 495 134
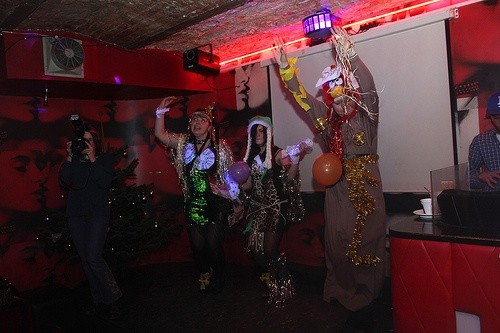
183 50 221 77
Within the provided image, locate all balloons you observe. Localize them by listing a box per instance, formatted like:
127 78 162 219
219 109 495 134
230 160 251 185
312 154 343 188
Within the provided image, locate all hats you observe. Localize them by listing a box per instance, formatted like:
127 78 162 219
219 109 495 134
486 92 500 115
243 114 273 169
316 64 361 105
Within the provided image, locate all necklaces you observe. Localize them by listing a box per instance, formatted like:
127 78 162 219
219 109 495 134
197 139 205 145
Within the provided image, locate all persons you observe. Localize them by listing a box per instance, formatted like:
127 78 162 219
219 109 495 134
226 116 303 299
154 96 240 302
215 60 272 119
271 24 386 333
0 86 183 291
57 129 128 320
468 91 500 190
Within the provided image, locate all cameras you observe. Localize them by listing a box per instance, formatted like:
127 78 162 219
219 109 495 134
69 114 91 158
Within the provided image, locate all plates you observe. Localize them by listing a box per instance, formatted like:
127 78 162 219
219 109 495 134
412 209 432 219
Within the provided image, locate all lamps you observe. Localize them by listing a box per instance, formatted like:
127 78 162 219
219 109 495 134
302 10 332 38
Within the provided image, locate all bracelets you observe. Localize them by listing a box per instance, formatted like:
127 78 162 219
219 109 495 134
156 107 170 118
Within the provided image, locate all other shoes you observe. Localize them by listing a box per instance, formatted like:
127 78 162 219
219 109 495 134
191 267 213 295
108 295 127 319
259 273 274 296
85 302 107 316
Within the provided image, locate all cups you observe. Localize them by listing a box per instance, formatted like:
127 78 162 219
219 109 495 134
420 198 432 214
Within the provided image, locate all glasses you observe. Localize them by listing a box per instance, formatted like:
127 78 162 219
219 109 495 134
489 114 500 119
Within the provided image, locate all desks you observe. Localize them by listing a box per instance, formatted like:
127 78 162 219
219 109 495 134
389 214 500 333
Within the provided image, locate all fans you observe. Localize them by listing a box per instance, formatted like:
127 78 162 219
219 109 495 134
43 34 85 79
